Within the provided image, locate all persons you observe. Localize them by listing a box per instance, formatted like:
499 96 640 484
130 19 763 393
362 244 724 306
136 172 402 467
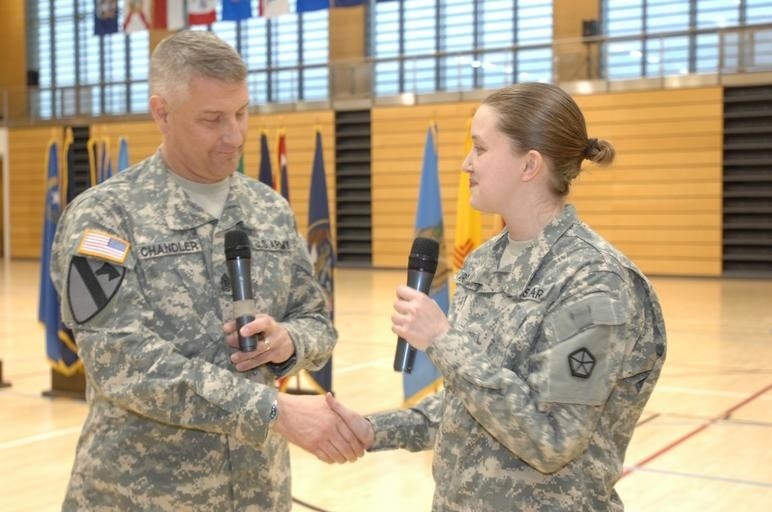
49 28 366 511
325 82 669 512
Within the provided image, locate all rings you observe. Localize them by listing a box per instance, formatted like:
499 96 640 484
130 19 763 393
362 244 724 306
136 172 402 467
262 338 272 351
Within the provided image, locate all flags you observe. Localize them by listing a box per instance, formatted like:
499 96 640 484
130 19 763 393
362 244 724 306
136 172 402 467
39 138 85 377
235 133 335 397
93 0 366 36
88 133 131 187
405 126 507 404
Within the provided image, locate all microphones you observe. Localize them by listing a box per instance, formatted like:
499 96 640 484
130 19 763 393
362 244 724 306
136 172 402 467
394 237 439 374
224 230 258 353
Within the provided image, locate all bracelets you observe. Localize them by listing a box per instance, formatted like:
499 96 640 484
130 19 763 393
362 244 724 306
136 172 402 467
267 399 280 428
